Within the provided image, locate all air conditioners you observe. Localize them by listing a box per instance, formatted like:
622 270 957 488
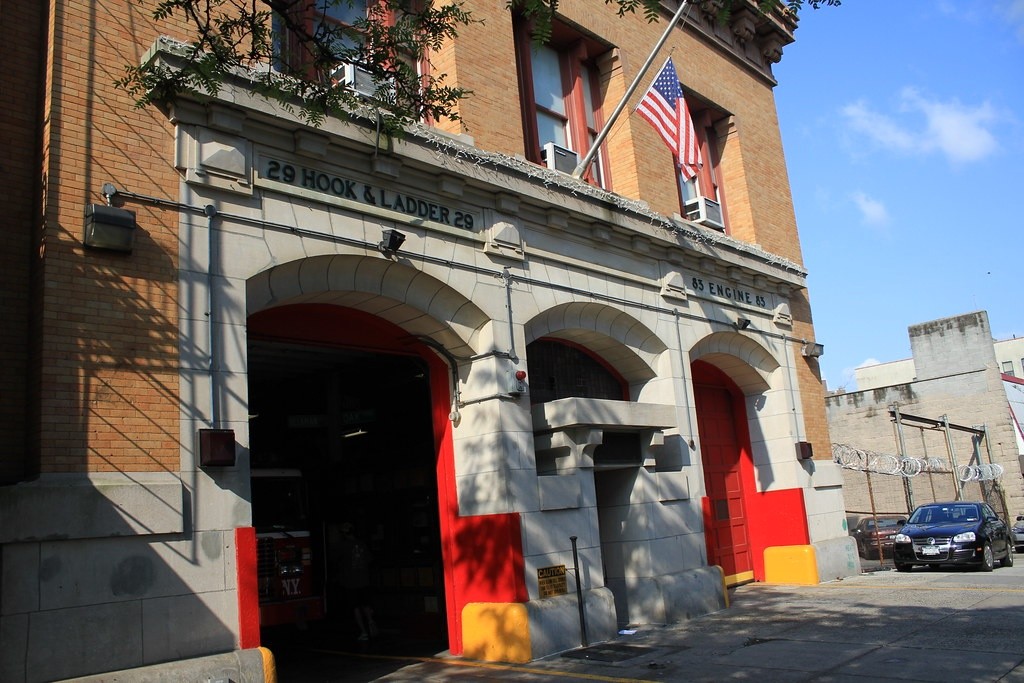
329 57 397 107
540 141 581 177
683 197 725 229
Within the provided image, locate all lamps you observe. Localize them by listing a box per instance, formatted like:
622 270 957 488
377 229 406 254
803 343 824 358
737 318 750 330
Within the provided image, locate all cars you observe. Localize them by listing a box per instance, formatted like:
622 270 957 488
892 500 1013 572
850 516 907 560
1012 516 1024 554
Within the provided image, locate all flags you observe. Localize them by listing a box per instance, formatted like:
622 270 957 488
636 55 704 185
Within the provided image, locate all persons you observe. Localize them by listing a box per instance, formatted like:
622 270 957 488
959 509 977 522
348 562 376 641
